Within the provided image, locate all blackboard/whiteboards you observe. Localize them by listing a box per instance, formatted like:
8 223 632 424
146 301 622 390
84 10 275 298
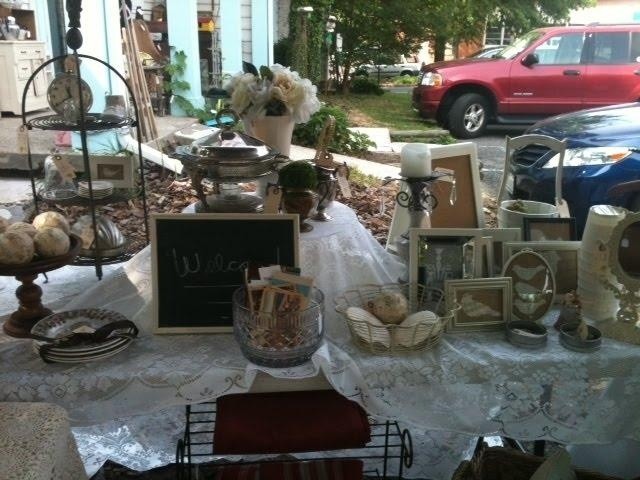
150 214 300 331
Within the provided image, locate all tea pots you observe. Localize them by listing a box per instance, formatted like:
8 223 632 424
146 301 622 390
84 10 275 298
0 15 21 40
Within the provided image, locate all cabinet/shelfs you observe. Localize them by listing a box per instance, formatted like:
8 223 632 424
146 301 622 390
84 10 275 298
20 54 152 272
177 393 417 480
0 39 57 116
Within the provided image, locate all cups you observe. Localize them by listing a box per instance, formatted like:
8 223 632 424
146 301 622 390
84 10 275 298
17 30 27 40
576 204 626 323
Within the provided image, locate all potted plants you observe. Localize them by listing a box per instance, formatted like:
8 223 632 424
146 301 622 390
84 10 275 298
277 161 319 235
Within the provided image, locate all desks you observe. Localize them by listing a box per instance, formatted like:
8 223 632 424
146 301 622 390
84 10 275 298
0 194 640 480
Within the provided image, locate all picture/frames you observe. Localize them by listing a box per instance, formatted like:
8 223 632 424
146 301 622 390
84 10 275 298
89 155 134 189
396 141 585 336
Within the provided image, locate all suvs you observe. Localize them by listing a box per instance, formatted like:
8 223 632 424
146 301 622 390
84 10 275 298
411 22 640 138
349 50 421 78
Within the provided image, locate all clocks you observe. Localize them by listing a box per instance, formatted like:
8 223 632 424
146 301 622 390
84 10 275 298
47 60 94 117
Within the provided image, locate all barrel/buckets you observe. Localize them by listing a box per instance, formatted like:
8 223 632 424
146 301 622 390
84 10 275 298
496 132 569 229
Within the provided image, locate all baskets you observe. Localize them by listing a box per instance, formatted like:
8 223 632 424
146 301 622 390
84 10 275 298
333 281 462 355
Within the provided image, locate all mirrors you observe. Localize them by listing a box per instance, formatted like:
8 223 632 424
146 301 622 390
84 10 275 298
617 221 640 281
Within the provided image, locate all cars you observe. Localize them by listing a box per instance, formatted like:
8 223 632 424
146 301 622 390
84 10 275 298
503 99 640 279
467 45 507 59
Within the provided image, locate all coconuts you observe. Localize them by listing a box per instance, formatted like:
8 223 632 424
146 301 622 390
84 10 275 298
0 211 70 264
367 292 408 324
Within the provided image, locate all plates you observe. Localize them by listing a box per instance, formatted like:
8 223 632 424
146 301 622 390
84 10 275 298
29 306 135 365
39 188 78 201
78 180 114 200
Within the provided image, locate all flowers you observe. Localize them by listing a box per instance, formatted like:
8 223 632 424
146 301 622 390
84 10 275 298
222 58 320 122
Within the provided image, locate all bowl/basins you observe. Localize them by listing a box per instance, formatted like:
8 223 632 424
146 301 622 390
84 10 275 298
232 281 326 368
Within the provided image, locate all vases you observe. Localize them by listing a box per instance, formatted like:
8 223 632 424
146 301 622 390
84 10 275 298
305 162 338 221
240 112 294 156
